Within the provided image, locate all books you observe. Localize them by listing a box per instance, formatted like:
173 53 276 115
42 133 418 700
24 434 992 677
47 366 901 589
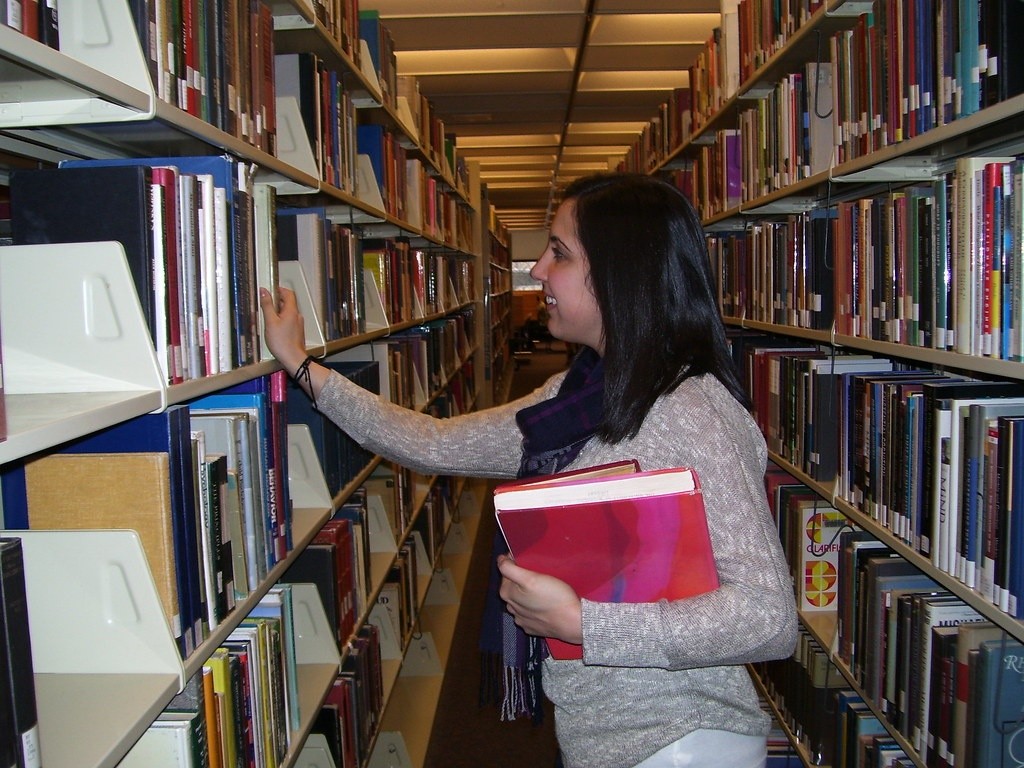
615 0 1023 768
495 459 718 661
1 0 507 768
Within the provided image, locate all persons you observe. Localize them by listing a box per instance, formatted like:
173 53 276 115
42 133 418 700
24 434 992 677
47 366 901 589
260 172 799 767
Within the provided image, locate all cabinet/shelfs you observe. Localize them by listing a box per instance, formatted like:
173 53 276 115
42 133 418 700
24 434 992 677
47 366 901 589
605 2 1024 768
0 0 517 768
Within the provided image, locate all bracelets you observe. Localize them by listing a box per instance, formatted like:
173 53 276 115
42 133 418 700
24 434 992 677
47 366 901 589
293 355 315 409
